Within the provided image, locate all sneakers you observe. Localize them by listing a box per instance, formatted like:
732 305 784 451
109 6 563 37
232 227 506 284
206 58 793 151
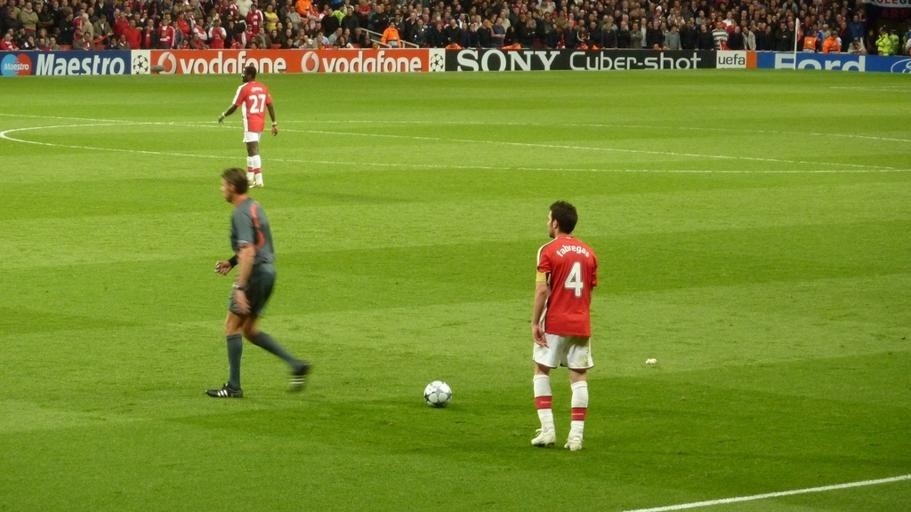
564 437 583 452
207 383 243 398
249 182 263 188
531 428 556 447
285 360 313 392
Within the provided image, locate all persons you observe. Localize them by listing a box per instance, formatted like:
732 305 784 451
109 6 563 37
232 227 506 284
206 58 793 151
530 199 598 453
207 167 314 398
218 66 279 189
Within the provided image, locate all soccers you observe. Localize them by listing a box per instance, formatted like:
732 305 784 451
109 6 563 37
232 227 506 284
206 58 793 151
424 380 453 408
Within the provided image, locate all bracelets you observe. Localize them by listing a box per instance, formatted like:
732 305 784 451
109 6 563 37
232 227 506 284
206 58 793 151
221 112 226 119
270 123 279 127
232 282 248 291
